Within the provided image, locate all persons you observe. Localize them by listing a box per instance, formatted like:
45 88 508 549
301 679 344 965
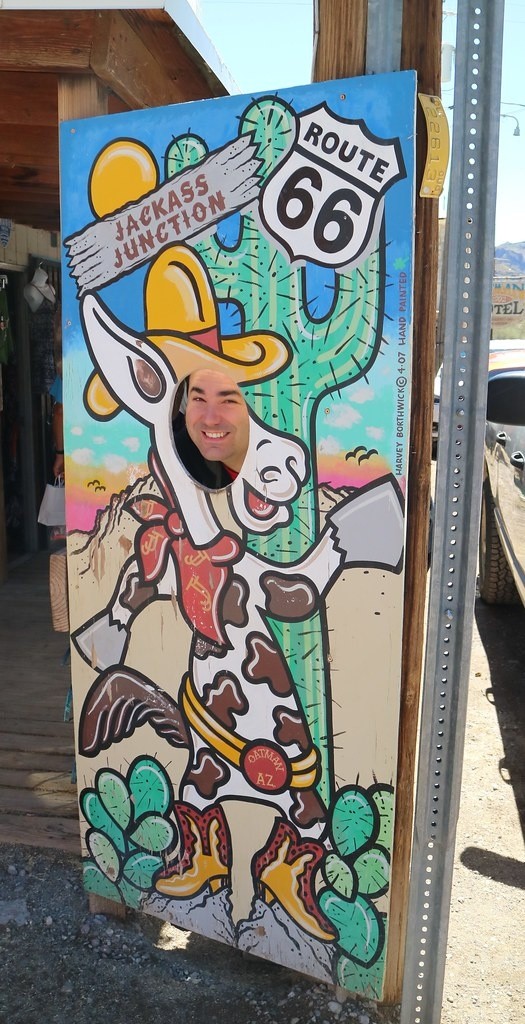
46 333 65 478
173 370 250 488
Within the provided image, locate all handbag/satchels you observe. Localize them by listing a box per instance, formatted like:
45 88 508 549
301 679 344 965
38 473 65 526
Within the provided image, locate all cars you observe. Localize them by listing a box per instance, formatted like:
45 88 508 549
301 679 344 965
432 338 525 460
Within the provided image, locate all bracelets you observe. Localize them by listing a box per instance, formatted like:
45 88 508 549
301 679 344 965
55 449 64 454
